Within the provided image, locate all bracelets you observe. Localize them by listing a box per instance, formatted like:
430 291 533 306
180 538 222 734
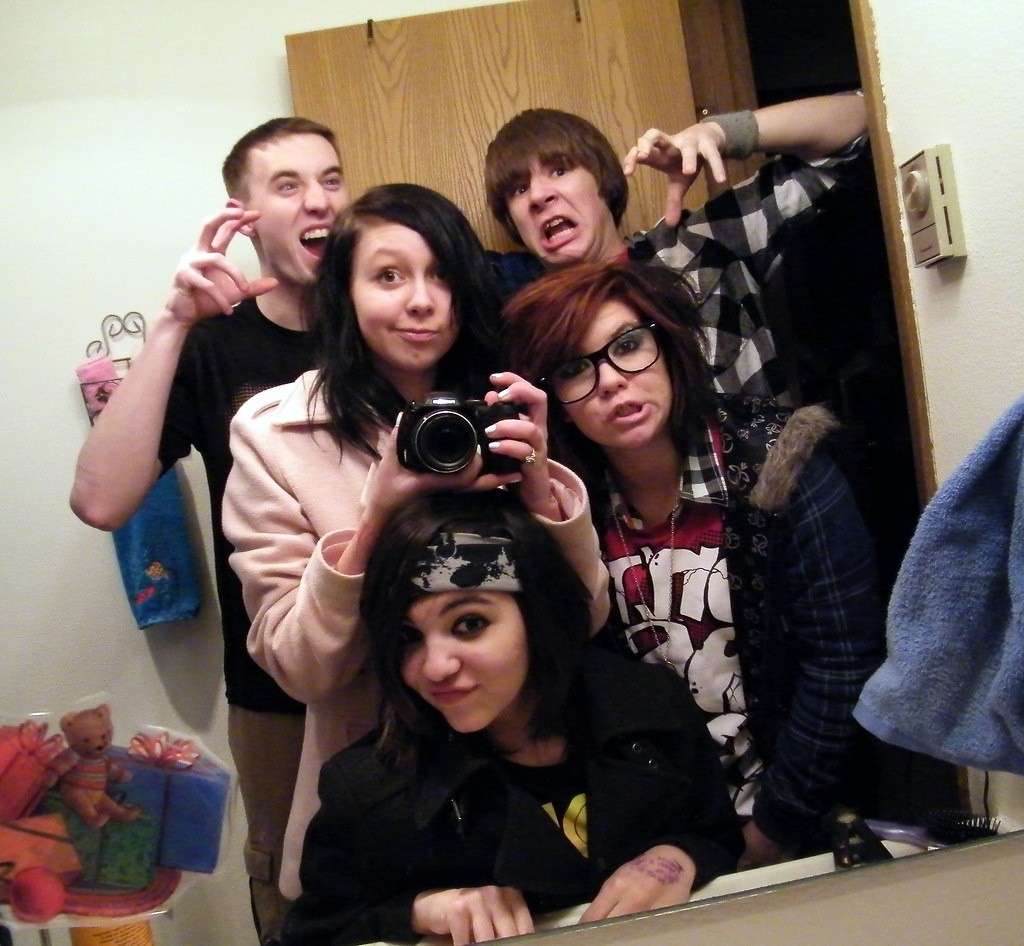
701 109 758 162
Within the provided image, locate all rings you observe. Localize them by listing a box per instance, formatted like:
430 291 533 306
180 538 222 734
521 448 537 467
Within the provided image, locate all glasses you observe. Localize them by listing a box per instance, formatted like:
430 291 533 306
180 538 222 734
541 321 660 403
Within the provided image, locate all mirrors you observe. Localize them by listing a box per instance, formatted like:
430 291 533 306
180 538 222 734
0 0 1024 945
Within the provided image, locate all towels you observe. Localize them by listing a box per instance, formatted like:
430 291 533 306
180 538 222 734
113 466 205 632
850 391 1024 776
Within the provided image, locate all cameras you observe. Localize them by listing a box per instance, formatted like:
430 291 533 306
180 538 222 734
405 396 519 473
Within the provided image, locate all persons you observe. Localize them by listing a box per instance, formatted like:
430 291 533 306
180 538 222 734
70 118 352 946
283 484 746 946
220 182 608 899
502 262 887 870
485 92 868 408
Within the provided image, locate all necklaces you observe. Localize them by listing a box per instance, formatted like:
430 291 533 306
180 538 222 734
608 463 683 671
